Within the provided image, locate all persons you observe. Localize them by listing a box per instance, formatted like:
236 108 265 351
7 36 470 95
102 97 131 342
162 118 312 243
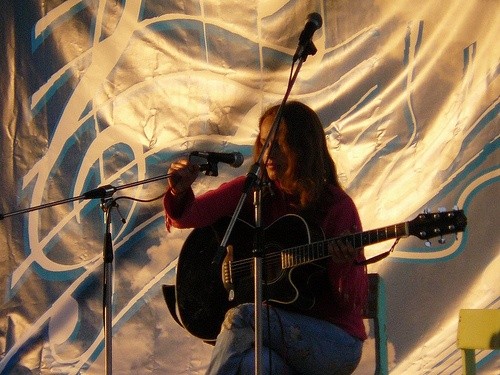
163 101 368 375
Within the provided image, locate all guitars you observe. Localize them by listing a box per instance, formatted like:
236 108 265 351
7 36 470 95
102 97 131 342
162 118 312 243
175 205 468 340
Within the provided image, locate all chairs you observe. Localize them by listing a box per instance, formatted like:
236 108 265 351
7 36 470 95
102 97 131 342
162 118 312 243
456 308 500 375
362 272 389 375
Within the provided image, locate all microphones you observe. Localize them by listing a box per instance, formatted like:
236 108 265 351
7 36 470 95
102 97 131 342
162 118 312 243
292 12 323 60
193 151 244 168
